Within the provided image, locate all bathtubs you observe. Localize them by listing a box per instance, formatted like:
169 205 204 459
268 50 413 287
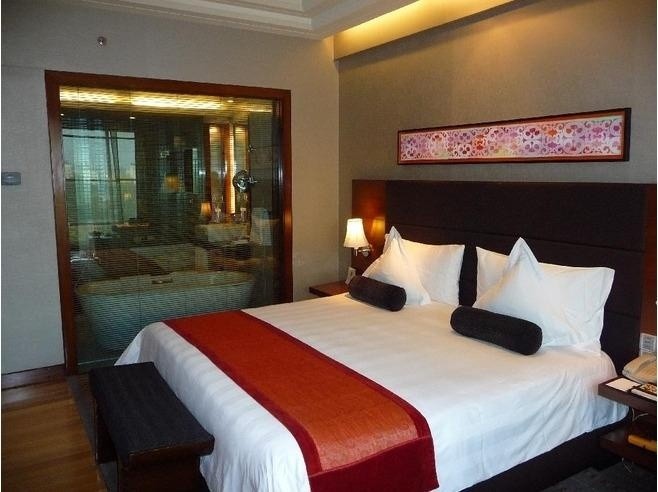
74 272 257 352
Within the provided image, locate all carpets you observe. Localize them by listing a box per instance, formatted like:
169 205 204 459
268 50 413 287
64 374 656 491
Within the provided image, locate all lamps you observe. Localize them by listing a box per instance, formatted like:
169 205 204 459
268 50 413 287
342 217 372 259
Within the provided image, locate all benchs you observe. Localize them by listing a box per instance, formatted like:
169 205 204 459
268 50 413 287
87 360 214 492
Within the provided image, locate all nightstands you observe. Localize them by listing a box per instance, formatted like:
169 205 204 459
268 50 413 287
597 376 656 475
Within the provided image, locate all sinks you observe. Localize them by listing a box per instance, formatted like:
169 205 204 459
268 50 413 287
195 224 249 241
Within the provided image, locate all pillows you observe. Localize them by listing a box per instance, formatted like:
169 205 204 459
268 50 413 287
348 223 618 357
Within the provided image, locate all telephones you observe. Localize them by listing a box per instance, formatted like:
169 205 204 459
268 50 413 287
622 354 657 385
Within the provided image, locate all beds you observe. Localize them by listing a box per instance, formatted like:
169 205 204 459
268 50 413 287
142 178 656 492
